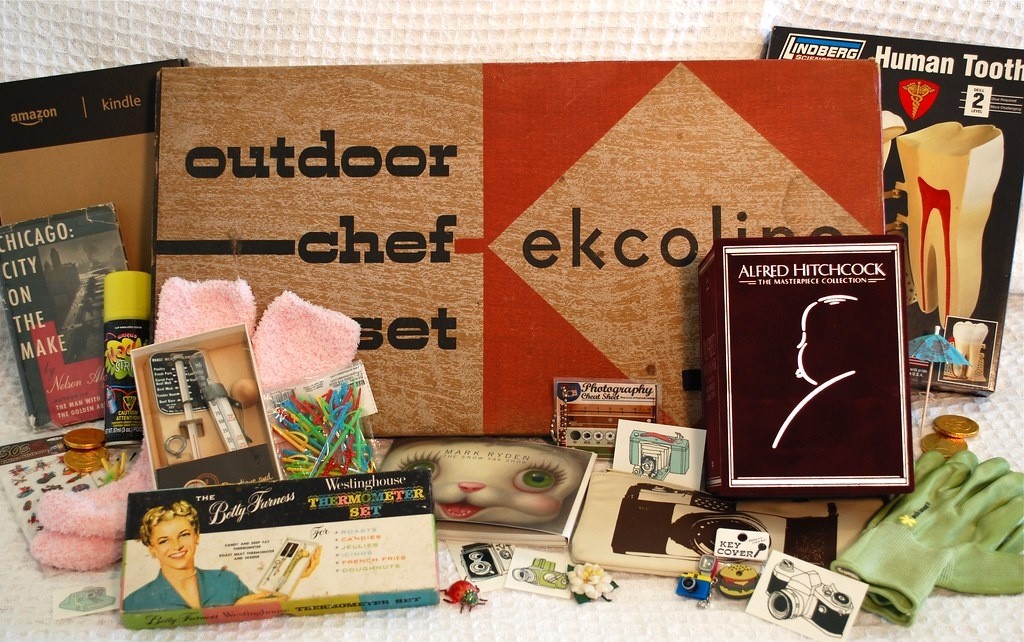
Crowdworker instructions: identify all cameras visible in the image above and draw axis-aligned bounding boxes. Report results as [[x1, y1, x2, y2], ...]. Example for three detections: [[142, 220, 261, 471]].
[[676, 576, 710, 599]]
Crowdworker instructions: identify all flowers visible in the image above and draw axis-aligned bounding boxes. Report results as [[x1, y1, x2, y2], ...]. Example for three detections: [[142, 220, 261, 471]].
[[564, 561, 614, 602]]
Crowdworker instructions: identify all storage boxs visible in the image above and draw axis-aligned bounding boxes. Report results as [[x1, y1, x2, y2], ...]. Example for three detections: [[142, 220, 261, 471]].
[[120, 468, 440, 630], [698, 234, 915, 497], [130, 321, 283, 490]]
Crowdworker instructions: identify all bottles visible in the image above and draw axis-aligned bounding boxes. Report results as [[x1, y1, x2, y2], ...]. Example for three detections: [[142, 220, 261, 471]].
[[103, 271, 155, 447]]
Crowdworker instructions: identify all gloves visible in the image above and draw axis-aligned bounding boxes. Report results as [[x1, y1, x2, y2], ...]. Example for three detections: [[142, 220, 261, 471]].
[[828, 448, 1024, 627]]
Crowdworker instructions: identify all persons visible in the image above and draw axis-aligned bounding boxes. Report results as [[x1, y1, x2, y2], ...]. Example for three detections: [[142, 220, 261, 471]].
[[121, 502, 320, 614]]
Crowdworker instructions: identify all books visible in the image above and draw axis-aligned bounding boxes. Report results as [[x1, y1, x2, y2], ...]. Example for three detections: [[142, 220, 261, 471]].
[[0, 203, 134, 432], [376, 435, 596, 549], [0, 59, 884, 456], [763, 25, 1024, 398]]
